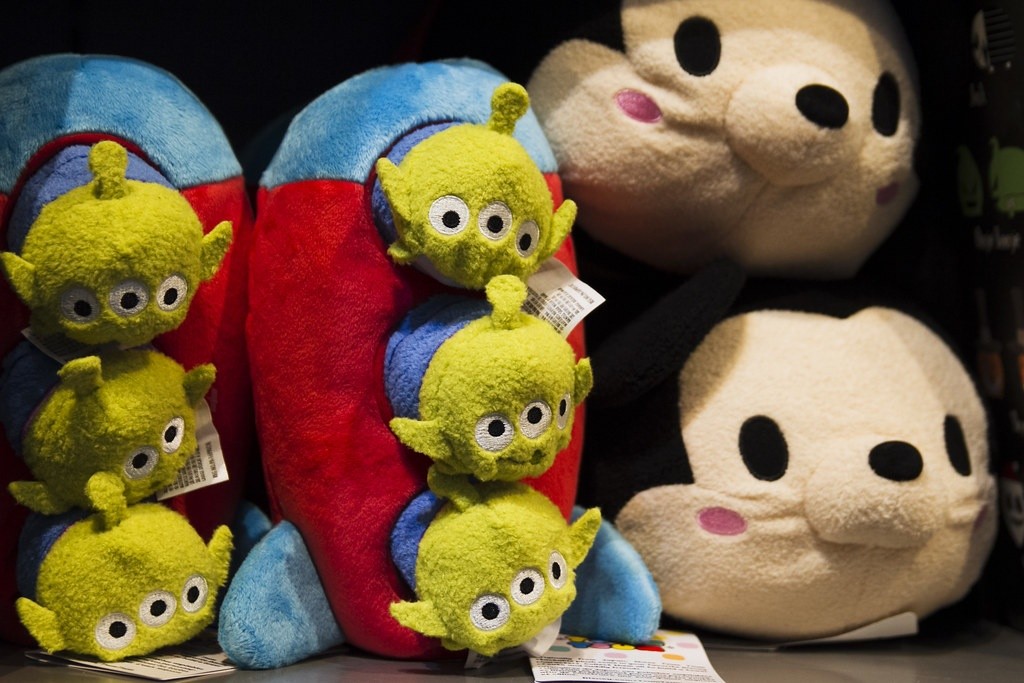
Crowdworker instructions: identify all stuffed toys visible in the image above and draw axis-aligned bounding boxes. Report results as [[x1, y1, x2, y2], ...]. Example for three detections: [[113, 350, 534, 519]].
[[574, 281, 1000, 639], [218, 57, 663, 667], [1, 50, 248, 658], [517, 0, 931, 280]]
